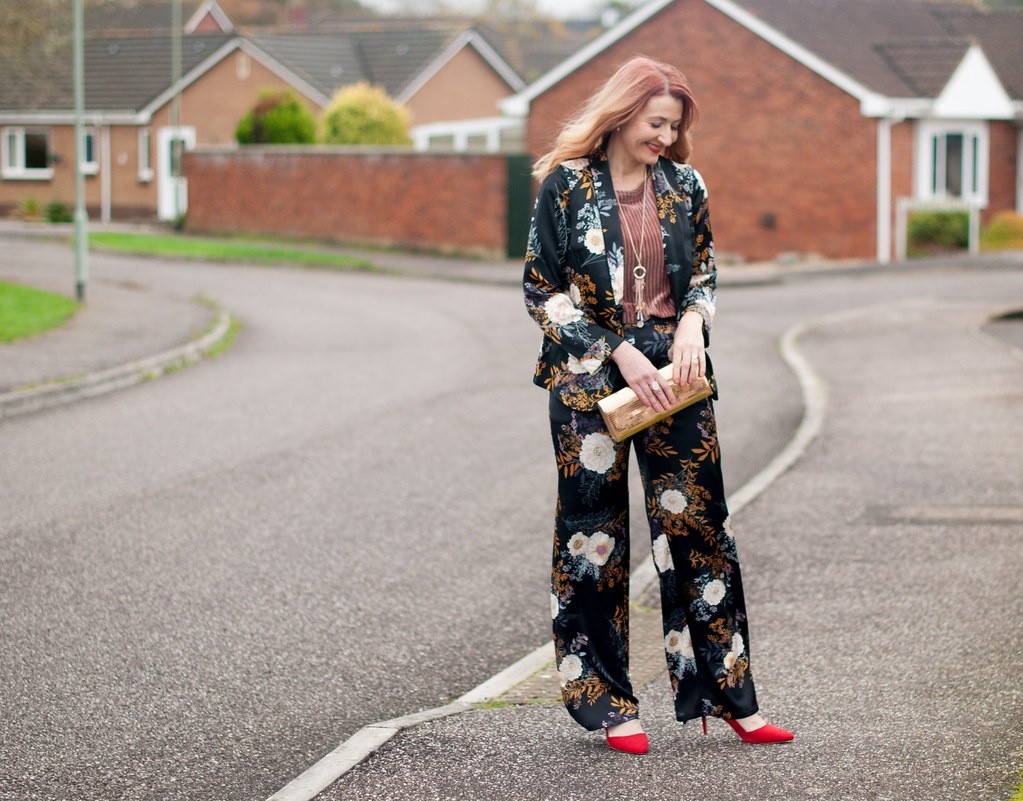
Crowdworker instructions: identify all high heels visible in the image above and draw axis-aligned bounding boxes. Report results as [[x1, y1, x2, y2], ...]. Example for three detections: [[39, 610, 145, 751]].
[[605, 723, 649, 755], [702, 715, 794, 743]]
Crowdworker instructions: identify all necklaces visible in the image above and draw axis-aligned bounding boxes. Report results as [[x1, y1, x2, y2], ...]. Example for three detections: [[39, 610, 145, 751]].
[[613, 166, 648, 322]]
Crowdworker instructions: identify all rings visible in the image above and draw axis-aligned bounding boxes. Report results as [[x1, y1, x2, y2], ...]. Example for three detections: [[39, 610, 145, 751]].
[[691, 355, 699, 360], [649, 381, 661, 391]]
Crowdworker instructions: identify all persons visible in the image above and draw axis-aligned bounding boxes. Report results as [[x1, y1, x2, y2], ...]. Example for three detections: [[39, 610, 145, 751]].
[[523, 56, 794, 754]]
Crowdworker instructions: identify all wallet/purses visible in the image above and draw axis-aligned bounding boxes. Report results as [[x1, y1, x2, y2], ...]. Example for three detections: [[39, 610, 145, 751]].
[[597, 361, 713, 443]]
